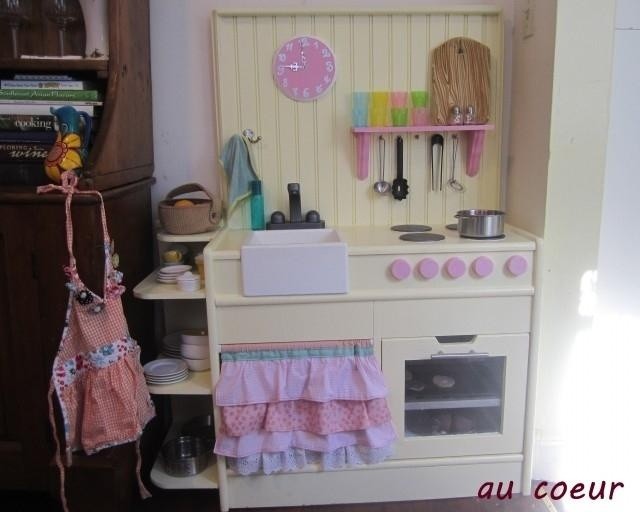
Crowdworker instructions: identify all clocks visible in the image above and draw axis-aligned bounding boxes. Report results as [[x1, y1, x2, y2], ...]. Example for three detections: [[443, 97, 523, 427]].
[[271, 35, 337, 102]]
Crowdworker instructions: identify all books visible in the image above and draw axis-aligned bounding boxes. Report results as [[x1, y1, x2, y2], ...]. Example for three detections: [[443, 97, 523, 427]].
[[0, 69, 106, 188]]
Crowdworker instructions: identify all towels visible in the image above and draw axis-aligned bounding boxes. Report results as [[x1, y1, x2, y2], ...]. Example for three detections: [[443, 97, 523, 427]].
[[215, 133, 264, 224]]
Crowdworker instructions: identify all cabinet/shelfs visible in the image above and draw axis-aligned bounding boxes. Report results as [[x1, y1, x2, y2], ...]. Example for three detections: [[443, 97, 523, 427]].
[[0, 179, 175, 509], [134, 219, 216, 493], [0, 0, 152, 188]]
